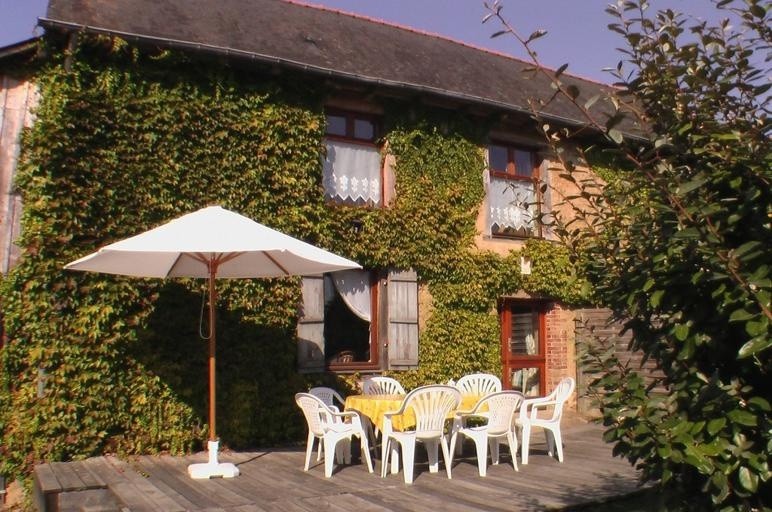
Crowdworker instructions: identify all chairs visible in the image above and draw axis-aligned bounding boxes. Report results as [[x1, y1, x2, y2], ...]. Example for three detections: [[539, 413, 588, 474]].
[[294, 392, 374, 479], [381, 384, 462, 484], [450, 391, 525, 476], [310, 387, 345, 462], [363, 378, 406, 394], [455, 375, 502, 394], [514, 377, 576, 466]]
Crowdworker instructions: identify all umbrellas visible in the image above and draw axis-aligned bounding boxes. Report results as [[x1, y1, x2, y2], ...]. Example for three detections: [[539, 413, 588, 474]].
[[60, 206, 363, 482]]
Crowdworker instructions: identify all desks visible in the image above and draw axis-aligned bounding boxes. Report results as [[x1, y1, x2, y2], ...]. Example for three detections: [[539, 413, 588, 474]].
[[342, 395, 503, 475]]
